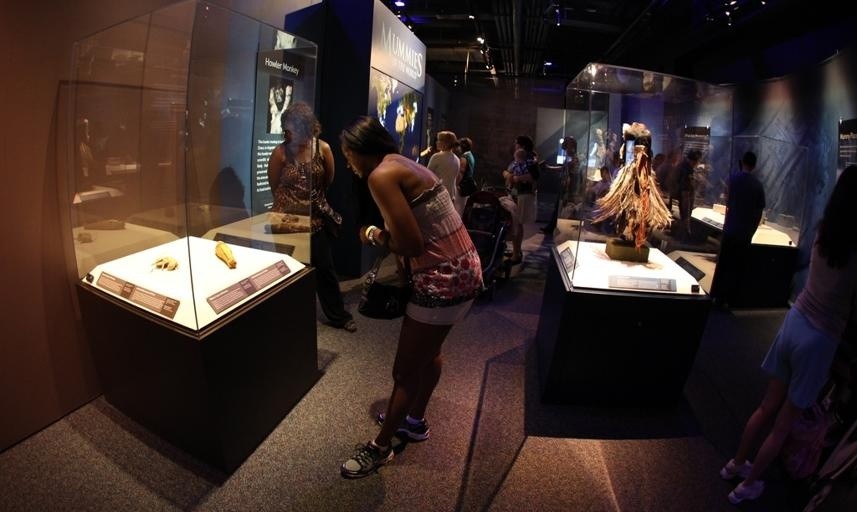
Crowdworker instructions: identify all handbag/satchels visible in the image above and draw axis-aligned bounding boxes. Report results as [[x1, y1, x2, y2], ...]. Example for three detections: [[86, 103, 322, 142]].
[[351, 243, 420, 318]]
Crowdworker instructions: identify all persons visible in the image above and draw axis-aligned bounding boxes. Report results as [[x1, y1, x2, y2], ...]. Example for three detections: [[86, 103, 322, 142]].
[[267, 101, 357, 333], [415, 131, 702, 266], [718, 152, 765, 300], [339, 115, 485, 479], [720, 164, 857, 503]]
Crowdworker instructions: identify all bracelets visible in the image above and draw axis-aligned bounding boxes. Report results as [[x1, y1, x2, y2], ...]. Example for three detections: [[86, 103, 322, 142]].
[[365, 225, 377, 246]]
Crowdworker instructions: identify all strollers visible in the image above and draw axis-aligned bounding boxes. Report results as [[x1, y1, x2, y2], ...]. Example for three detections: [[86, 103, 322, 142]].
[[462, 190, 514, 301]]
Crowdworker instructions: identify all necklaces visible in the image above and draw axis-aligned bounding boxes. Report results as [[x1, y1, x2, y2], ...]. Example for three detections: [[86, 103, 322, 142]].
[[294, 143, 307, 176]]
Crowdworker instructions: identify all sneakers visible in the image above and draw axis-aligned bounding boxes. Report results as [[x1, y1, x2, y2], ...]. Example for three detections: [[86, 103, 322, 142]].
[[721, 459, 771, 506], [378, 410, 432, 440], [341, 440, 396, 480], [335, 313, 360, 334], [510, 249, 526, 267]]
